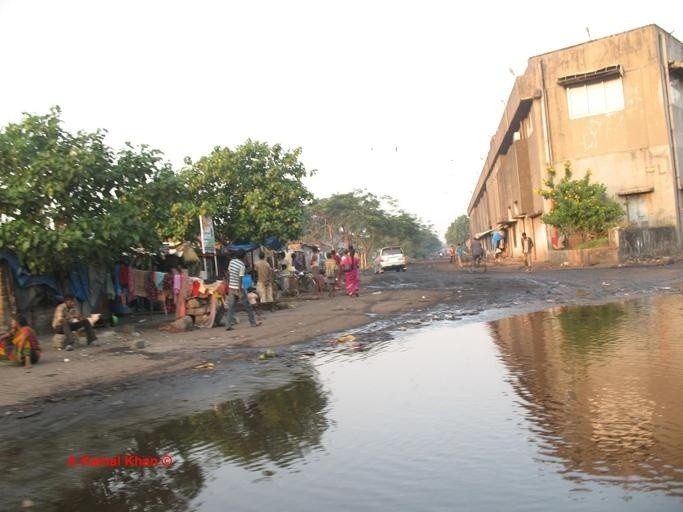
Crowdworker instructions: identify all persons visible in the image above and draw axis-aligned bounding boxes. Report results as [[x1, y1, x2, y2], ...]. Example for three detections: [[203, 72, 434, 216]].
[[457, 244, 462, 265], [521, 233, 533, 271], [472, 238, 483, 268], [225, 246, 360, 330], [52, 293, 100, 351], [450, 245, 455, 262], [495, 247, 502, 260], [0, 312, 41, 369]]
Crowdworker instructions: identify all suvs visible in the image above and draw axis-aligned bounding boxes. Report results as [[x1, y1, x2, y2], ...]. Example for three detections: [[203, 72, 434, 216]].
[[372, 245, 407, 274]]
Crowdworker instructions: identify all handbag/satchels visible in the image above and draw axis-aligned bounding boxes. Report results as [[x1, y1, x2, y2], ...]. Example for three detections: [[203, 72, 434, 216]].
[[343, 264, 352, 272]]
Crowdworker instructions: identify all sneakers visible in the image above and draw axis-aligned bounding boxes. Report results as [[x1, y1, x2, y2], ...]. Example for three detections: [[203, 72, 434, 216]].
[[92, 339, 100, 346], [66, 344, 74, 351]]
